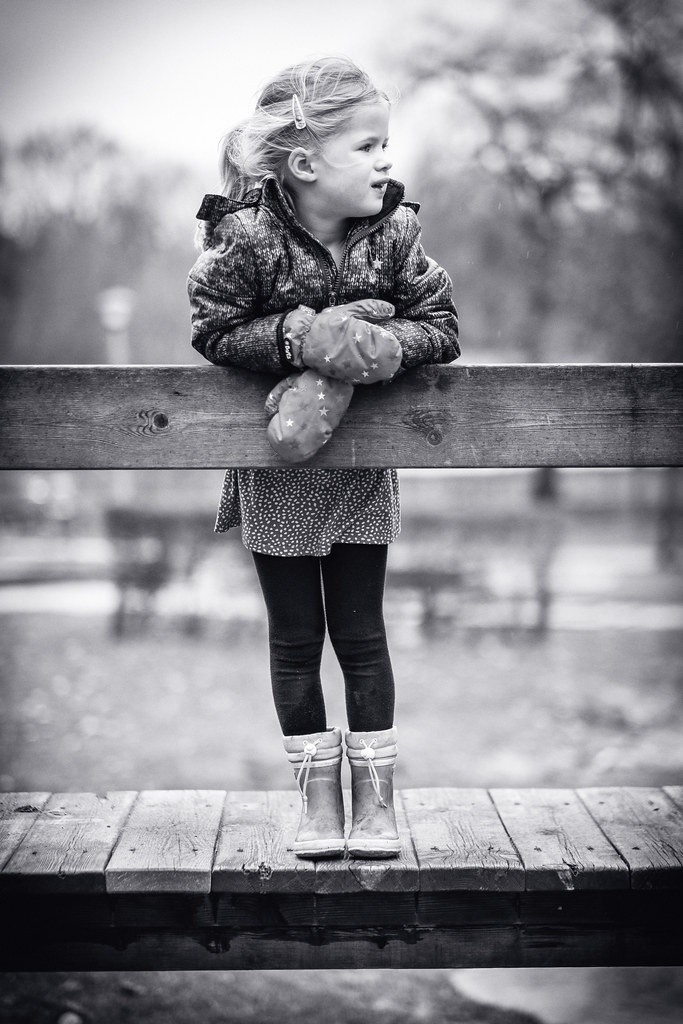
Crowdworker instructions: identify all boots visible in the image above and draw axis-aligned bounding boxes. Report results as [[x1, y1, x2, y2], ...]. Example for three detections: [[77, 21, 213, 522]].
[[344, 726, 402, 857], [282, 727, 347, 857]]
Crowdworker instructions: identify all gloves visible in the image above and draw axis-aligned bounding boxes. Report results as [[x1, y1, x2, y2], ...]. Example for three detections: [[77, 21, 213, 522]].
[[282, 298, 403, 385], [263, 369, 354, 464]]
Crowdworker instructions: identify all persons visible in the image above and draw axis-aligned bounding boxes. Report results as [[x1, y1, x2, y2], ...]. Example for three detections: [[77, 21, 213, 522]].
[[186, 57, 463, 860]]
